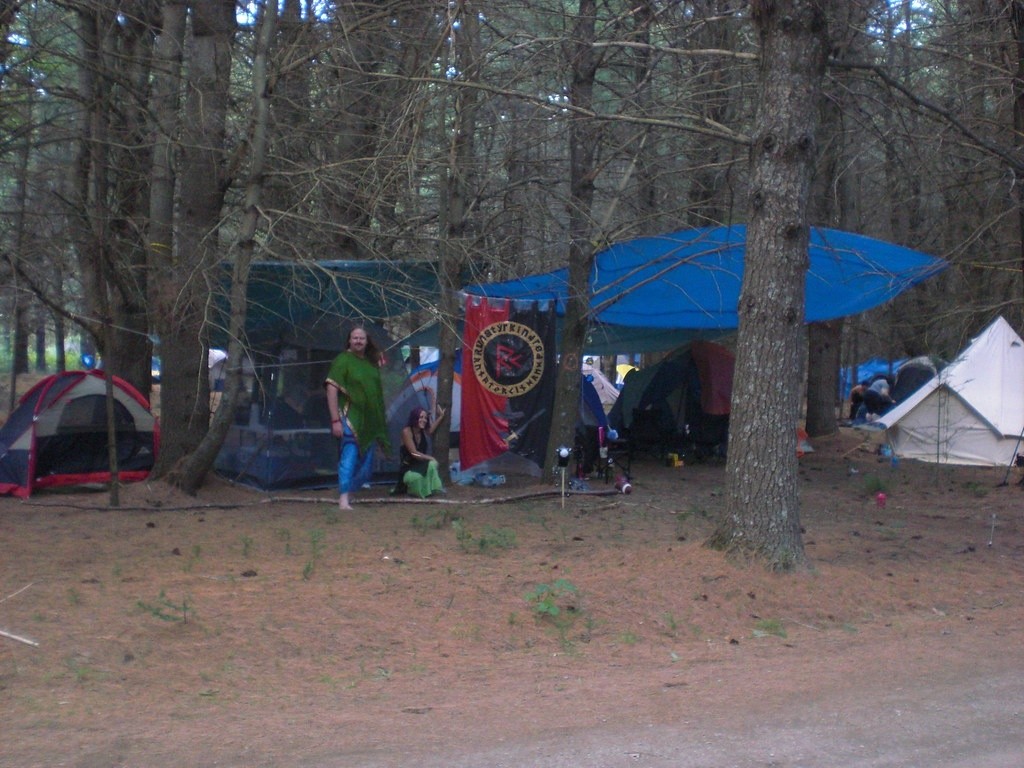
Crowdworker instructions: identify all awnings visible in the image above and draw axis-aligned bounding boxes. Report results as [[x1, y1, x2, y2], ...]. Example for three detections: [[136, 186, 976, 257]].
[[460, 221, 953, 330]]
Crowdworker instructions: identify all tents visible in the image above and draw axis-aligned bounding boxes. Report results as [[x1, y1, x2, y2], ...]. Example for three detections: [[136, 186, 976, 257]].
[[897, 355, 952, 390], [150, 334, 814, 491], [0, 366, 167, 500], [860, 316, 1024, 466]]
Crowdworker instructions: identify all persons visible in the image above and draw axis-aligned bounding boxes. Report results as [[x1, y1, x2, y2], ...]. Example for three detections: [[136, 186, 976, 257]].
[[848, 376, 897, 422], [325, 324, 389, 510], [390, 405, 446, 497]]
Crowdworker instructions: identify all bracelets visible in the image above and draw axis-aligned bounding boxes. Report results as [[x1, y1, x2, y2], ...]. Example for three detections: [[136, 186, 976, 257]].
[[332, 418, 341, 423]]
